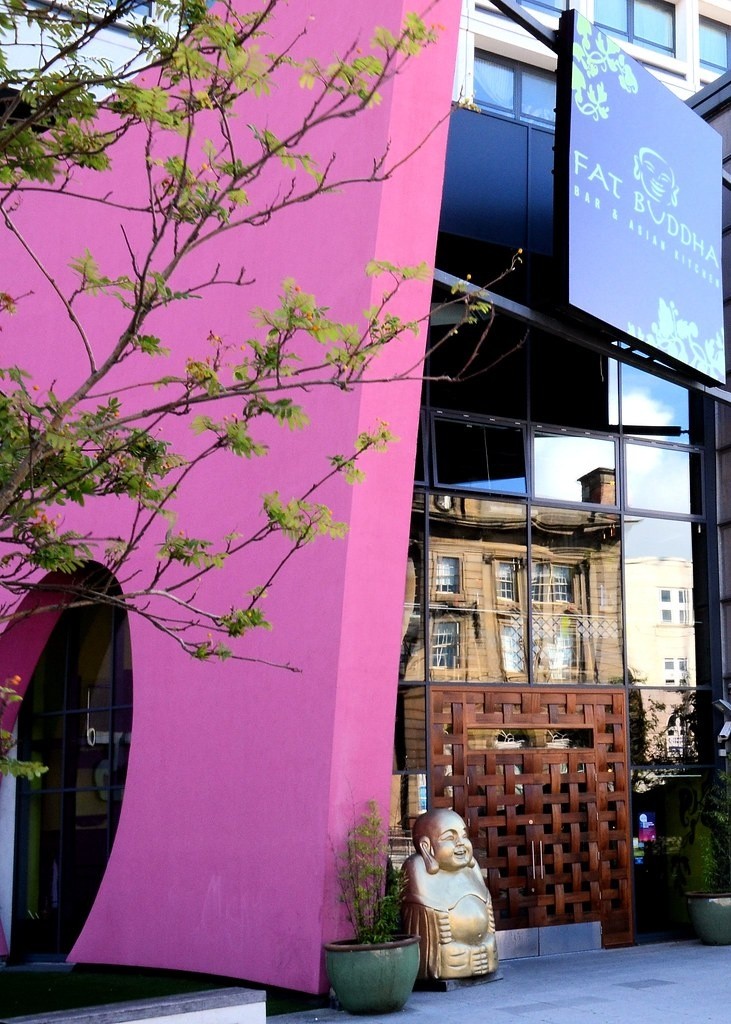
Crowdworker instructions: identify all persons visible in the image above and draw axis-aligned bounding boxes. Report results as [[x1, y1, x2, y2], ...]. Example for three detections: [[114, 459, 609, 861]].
[[398, 808, 500, 979]]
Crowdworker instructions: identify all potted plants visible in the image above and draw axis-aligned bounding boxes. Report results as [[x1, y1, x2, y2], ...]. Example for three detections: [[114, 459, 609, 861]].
[[319, 796, 422, 1017], [685, 768, 731, 946]]
